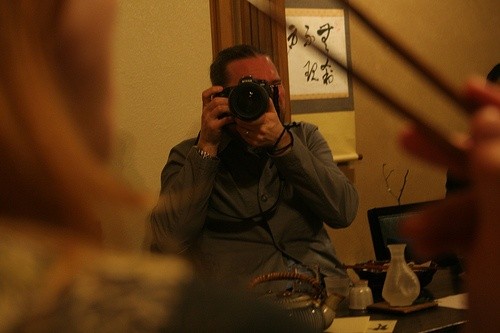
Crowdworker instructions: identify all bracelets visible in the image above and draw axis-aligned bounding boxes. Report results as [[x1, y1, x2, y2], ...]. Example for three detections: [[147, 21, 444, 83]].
[[268, 131, 294, 155], [191, 145, 217, 161]]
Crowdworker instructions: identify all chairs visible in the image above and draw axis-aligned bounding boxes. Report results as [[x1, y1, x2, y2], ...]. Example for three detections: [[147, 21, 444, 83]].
[[367, 199, 464, 295]]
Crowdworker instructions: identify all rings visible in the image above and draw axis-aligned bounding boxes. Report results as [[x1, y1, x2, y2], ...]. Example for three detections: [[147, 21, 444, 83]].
[[242, 129, 248, 136]]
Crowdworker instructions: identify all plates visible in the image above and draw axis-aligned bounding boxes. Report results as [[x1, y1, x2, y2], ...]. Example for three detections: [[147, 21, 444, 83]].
[[366, 300, 438, 314]]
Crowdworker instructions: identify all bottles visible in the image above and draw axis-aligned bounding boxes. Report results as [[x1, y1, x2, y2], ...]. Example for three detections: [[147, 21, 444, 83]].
[[382, 243, 420, 307], [348, 280, 374, 312]]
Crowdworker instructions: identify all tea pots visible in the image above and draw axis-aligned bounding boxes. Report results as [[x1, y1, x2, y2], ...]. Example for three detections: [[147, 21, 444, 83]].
[[244, 272, 347, 333]]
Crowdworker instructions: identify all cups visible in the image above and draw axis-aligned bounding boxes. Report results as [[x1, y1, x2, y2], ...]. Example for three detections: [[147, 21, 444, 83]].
[[322, 275, 347, 298]]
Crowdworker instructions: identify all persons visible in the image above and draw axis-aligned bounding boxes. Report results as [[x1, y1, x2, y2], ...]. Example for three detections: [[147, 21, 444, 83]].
[[400, 75, 500, 333], [150, 44, 359, 298]]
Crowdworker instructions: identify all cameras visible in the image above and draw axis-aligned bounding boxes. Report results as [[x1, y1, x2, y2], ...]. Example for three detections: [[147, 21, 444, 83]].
[[213, 75, 276, 128]]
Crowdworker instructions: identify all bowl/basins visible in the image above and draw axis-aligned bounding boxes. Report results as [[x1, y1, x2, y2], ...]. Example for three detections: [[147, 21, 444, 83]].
[[353, 260, 438, 291]]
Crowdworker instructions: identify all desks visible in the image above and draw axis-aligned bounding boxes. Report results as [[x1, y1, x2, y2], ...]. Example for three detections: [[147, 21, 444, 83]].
[[338, 263, 470, 333]]
